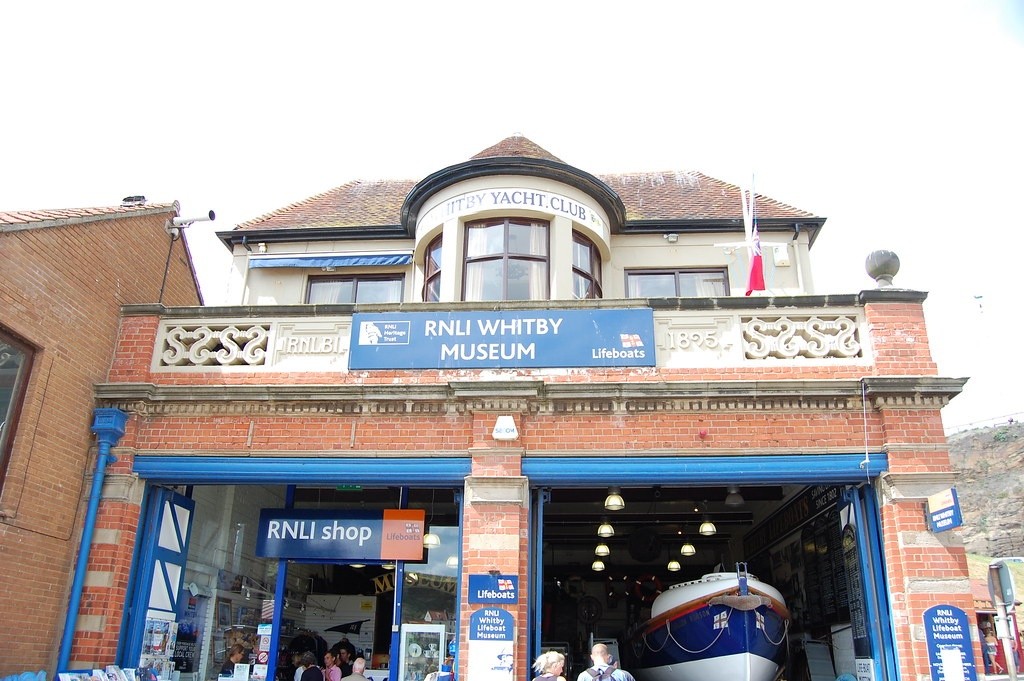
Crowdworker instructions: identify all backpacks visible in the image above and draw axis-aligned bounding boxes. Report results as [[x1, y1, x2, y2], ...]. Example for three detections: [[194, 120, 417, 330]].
[[585, 667, 617, 681]]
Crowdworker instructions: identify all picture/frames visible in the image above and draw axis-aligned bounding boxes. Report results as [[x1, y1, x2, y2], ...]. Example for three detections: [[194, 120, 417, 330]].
[[215, 596, 234, 634], [211, 635, 227, 668]]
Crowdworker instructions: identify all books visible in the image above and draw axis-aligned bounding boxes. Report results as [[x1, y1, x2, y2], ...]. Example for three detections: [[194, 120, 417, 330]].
[[58, 617, 178, 681]]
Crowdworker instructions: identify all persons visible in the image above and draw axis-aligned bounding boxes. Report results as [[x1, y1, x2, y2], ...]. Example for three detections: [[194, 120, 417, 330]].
[[529, 650, 567, 681], [979, 628, 1024, 675], [217, 638, 371, 681], [576, 643, 635, 681]]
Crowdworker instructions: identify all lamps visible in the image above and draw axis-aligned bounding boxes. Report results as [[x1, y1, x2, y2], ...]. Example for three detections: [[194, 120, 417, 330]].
[[592, 556, 605, 571], [667, 556, 681, 571], [604, 487, 625, 511], [597, 518, 616, 538], [680, 538, 696, 556], [594, 540, 610, 557], [698, 516, 717, 536]]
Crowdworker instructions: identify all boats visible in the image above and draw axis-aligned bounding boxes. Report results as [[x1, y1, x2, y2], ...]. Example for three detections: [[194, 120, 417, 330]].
[[629, 560, 794, 681]]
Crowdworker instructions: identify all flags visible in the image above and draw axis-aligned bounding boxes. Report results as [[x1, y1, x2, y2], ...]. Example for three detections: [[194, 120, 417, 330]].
[[745, 174, 765, 296]]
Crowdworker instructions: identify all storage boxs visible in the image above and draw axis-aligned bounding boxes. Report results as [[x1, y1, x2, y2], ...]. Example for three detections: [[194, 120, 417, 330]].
[[397, 623, 448, 681]]
